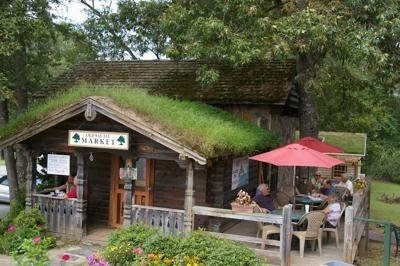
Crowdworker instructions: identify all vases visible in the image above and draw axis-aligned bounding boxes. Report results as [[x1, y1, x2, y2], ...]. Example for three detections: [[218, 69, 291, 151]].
[[231, 202, 254, 215]]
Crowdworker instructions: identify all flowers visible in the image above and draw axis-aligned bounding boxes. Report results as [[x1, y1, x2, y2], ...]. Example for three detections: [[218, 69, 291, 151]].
[[353, 179, 367, 187], [235, 188, 252, 205]]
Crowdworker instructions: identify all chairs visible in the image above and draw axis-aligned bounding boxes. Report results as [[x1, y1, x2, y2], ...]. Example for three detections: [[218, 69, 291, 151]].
[[254, 175, 356, 259]]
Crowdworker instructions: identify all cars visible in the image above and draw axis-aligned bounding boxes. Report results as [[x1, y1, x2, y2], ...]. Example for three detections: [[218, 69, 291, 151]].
[[0, 169, 47, 204]]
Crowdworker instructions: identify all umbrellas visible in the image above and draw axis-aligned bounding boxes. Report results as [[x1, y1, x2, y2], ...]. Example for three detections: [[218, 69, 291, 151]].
[[293, 136, 344, 181], [245, 143, 344, 214]]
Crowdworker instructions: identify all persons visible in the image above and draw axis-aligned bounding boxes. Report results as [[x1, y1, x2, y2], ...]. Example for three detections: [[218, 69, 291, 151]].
[[294, 171, 354, 231], [252, 183, 282, 240], [54, 175, 73, 194], [64, 176, 77, 198]]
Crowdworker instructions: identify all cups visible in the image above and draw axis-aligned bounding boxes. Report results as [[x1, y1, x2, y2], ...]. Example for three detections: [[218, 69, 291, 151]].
[[304, 204, 310, 213]]
